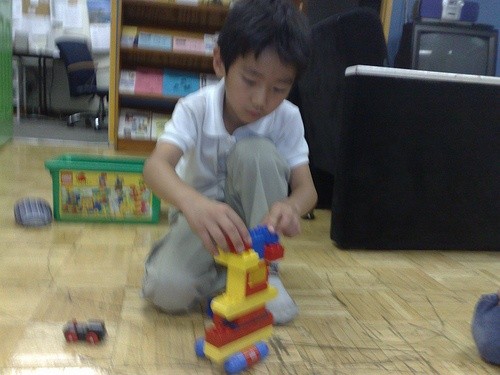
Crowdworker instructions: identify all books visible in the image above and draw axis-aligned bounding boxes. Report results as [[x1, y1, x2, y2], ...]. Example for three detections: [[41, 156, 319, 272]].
[[117, 24, 220, 143]]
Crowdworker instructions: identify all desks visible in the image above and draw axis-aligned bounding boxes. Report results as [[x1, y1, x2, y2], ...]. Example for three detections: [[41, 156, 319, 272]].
[[13, 49, 61, 119], [329, 64, 500, 251]]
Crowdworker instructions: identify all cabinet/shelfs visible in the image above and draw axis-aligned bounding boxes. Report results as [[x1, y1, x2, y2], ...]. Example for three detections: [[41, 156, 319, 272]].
[[108, 0, 229, 150]]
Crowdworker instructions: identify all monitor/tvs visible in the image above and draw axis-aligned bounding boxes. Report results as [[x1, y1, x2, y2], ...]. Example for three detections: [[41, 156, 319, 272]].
[[394, 22, 498, 76]]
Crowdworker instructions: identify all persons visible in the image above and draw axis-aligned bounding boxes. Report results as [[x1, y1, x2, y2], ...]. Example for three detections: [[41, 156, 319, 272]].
[[139, 0, 320, 325], [471, 289, 500, 367]]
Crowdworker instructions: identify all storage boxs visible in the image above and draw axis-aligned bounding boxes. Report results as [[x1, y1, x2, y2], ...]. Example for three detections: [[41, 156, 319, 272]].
[[14, 29, 48, 54], [44, 153, 160, 224]]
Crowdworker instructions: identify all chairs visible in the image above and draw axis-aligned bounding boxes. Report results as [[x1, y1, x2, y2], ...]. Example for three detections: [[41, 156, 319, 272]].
[[55, 36, 109, 132]]
